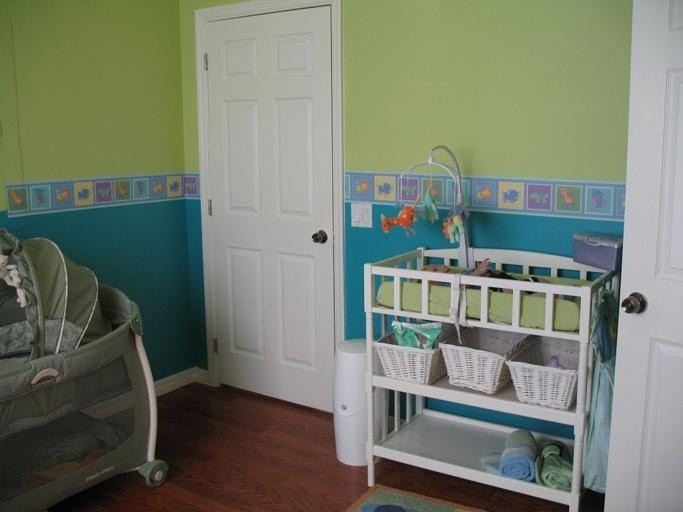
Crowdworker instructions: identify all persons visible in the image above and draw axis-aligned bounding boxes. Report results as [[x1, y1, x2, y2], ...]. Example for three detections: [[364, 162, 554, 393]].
[[417, 256, 550, 299]]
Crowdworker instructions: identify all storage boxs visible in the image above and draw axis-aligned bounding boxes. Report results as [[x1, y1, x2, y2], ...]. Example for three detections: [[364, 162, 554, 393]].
[[571, 233, 623, 272]]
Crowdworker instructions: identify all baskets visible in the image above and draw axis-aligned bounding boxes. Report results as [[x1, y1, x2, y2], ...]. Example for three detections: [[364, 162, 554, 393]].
[[438, 328, 530, 395], [505, 335, 578, 410], [373, 333, 444, 385]]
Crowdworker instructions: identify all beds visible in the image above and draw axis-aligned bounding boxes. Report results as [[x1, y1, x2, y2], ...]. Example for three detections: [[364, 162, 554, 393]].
[[363, 245, 622, 512]]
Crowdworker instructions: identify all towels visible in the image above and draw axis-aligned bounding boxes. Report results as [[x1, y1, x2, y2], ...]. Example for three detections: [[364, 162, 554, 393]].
[[498, 430, 540, 483], [534, 436, 573, 492]]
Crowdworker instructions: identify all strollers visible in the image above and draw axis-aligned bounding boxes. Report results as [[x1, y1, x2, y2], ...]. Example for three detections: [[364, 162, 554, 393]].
[[0, 227, 168, 511]]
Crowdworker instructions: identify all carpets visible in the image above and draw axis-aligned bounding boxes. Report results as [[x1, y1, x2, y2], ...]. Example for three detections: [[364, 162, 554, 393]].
[[345, 483, 487, 512]]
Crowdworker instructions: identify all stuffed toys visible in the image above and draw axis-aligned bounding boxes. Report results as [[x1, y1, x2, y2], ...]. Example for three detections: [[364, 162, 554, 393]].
[[378, 203, 421, 238], [420, 188, 440, 227], [440, 206, 470, 246]]
